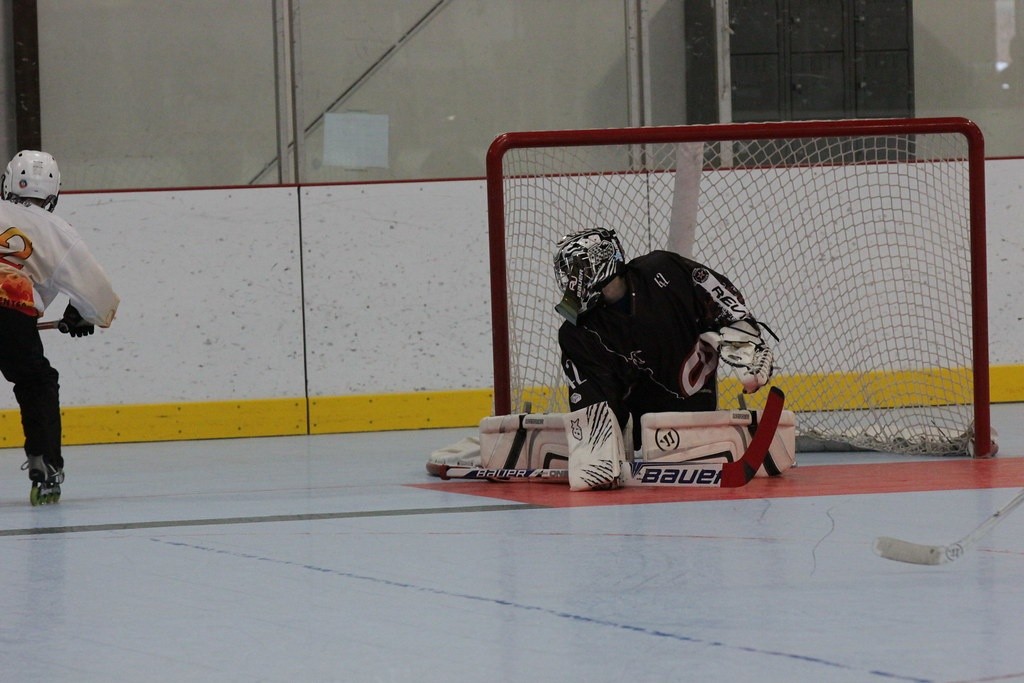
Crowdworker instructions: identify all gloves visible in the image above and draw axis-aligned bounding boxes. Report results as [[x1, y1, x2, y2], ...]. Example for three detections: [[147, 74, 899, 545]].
[[58, 300, 95, 338]]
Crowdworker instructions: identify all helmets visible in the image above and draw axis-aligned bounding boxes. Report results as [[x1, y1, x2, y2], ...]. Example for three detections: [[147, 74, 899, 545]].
[[0, 150, 61, 213], [554, 228, 625, 314]]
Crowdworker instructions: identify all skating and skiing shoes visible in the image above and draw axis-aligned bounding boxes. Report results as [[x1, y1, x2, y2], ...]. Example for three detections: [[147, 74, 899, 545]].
[[20, 456, 65, 505]]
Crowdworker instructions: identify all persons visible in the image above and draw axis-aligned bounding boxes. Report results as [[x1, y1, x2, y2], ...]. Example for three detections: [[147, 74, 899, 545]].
[[0, 150, 121, 505], [426, 227, 796, 492]]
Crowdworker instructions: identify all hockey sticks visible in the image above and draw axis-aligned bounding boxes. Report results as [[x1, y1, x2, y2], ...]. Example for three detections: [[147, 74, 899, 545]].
[[439, 385, 786, 490], [36, 320, 60, 330]]
